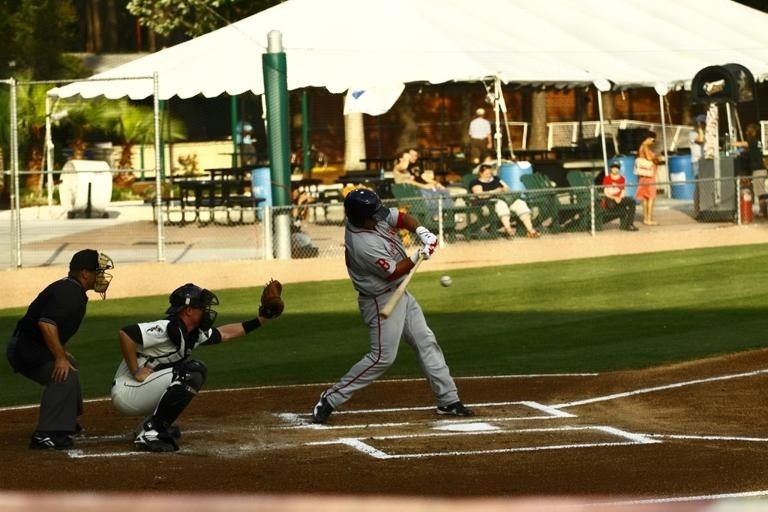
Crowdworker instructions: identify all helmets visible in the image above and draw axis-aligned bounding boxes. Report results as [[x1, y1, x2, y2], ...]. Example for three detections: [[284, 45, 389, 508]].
[[343, 189, 391, 229]]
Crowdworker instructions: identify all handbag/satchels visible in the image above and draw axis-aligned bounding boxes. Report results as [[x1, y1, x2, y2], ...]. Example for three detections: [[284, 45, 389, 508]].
[[633, 157, 661, 179]]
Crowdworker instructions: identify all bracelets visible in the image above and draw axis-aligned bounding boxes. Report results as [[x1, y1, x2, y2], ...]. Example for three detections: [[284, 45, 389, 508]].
[[132, 367, 140, 377], [410, 252, 419, 265], [242, 317, 262, 334]]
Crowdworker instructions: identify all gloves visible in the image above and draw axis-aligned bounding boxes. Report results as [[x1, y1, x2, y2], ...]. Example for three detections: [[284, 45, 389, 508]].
[[410, 227, 438, 266]]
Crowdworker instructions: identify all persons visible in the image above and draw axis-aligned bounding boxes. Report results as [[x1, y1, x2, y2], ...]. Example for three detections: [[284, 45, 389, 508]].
[[110, 277, 285, 452], [602, 162, 638, 231], [235, 107, 259, 167], [7, 249, 114, 451], [635, 134, 659, 225], [689, 114, 706, 179], [290, 111, 316, 173], [312, 188, 474, 424], [392, 108, 540, 239], [729, 123, 764, 176]]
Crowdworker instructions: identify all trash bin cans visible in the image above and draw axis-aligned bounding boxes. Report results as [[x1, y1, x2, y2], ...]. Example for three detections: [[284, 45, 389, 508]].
[[499, 161, 533, 200]]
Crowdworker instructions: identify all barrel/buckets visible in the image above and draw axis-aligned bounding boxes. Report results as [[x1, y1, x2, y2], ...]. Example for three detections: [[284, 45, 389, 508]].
[[499, 161, 533, 195]]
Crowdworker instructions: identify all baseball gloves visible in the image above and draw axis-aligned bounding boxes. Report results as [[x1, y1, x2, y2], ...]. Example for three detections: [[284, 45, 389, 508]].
[[261, 280, 284, 317]]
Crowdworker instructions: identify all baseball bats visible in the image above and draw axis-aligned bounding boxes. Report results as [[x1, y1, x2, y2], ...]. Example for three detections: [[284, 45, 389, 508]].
[[380, 254, 423, 319]]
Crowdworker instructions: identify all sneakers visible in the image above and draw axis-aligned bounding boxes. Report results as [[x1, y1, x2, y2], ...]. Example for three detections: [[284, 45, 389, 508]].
[[28, 432, 73, 450], [620, 225, 638, 232], [524, 230, 539, 238], [435, 400, 476, 421], [504, 231, 514, 239], [642, 219, 659, 226], [130, 421, 182, 454], [311, 388, 331, 423]]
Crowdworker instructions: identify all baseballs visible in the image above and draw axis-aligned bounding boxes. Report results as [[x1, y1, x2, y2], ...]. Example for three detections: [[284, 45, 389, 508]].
[[441, 276, 451, 286]]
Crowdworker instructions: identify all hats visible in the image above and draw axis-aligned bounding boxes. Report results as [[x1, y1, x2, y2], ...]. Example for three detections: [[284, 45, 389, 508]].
[[608, 161, 621, 168], [643, 131, 657, 139], [163, 282, 214, 314], [69, 248, 111, 273]]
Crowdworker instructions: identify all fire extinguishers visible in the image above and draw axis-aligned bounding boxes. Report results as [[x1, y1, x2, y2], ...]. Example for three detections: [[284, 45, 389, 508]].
[[740, 178, 752, 224]]
